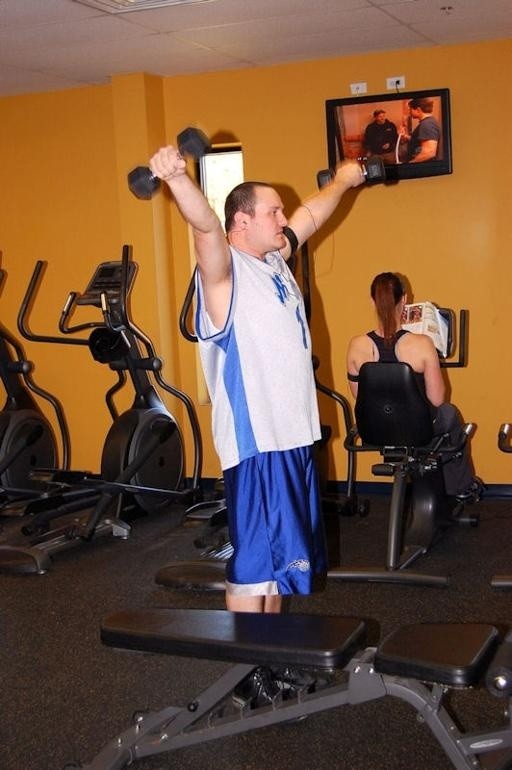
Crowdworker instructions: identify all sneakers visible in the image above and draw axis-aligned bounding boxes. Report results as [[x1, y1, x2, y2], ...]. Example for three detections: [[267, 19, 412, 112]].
[[232, 666, 309, 725], [455, 478, 482, 504], [273, 664, 336, 693]]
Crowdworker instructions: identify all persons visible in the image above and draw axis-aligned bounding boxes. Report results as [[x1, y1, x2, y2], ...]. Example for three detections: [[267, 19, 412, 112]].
[[149, 145, 366, 728], [361, 109, 398, 165], [346, 272, 483, 504], [400, 98, 440, 165]]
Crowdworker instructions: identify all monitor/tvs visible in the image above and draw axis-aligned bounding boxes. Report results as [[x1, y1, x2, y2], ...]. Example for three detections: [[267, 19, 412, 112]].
[[325, 87, 453, 182], [76, 260, 139, 306]]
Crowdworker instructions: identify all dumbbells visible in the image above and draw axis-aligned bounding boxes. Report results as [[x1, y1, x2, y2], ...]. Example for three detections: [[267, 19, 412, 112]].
[[128, 127, 212, 200], [317, 157, 386, 190]]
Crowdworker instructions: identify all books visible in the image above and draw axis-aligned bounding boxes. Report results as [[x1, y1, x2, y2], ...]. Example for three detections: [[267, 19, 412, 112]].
[[399, 301, 448, 358]]
